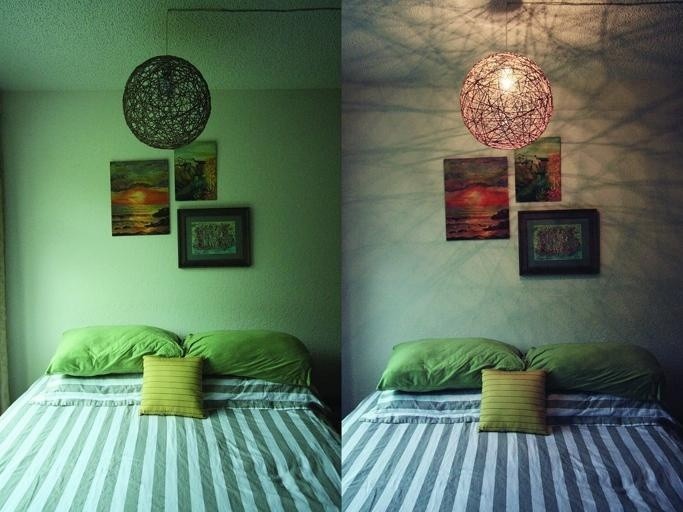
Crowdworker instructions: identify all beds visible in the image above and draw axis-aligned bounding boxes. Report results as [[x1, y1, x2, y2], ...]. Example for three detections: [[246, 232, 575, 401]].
[[0, 368, 341, 511], [342, 388, 683, 511]]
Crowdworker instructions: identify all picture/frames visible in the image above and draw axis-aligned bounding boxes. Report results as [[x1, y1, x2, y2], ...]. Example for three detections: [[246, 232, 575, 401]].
[[178, 208, 250, 267], [518, 209, 600, 276]]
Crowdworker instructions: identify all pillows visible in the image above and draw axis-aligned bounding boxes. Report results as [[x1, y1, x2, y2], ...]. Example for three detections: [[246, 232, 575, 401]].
[[47, 321, 315, 419], [377, 336, 667, 434]]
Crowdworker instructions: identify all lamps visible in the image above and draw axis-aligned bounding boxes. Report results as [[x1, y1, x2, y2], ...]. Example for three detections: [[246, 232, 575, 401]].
[[460, 0, 554, 152], [122, 0, 212, 151]]
